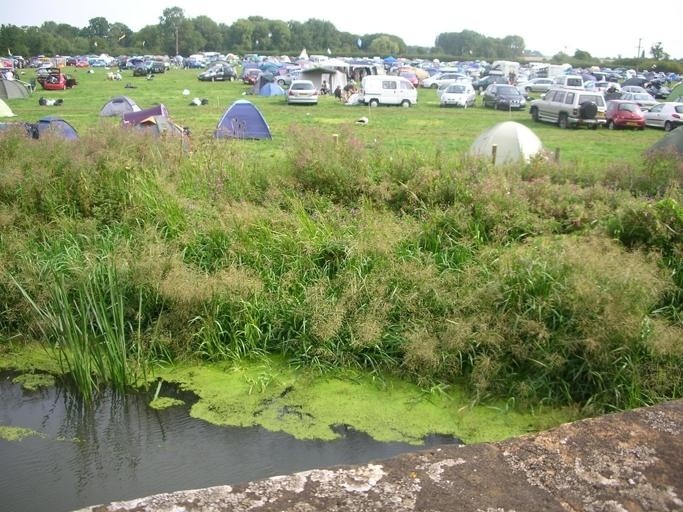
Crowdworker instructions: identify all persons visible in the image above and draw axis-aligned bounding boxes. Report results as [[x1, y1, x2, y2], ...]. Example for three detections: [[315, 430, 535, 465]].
[[107, 71, 122, 81], [320, 72, 358, 103]]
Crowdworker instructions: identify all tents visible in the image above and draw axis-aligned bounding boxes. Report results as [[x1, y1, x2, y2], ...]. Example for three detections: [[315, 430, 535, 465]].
[[214, 99, 271, 141], [467, 121, 548, 169], [259, 82, 284, 97], [0, 71, 79, 148], [641, 121, 683, 161], [99, 95, 188, 152]]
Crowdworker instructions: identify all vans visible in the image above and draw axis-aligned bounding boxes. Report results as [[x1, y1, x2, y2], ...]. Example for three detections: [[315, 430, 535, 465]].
[[143, 61, 164, 74], [360, 74, 417, 107]]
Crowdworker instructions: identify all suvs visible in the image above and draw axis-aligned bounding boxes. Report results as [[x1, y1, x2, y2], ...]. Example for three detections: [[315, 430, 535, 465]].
[[132, 64, 147, 76]]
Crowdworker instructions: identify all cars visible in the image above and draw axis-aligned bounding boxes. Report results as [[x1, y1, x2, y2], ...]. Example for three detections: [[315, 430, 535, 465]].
[[420, 61, 683, 131], [275, 69, 302, 86], [242, 69, 264, 84], [286, 79, 318, 104], [184, 57, 211, 68], [37, 67, 77, 90], [197, 66, 237, 82], [64, 55, 170, 70]]
